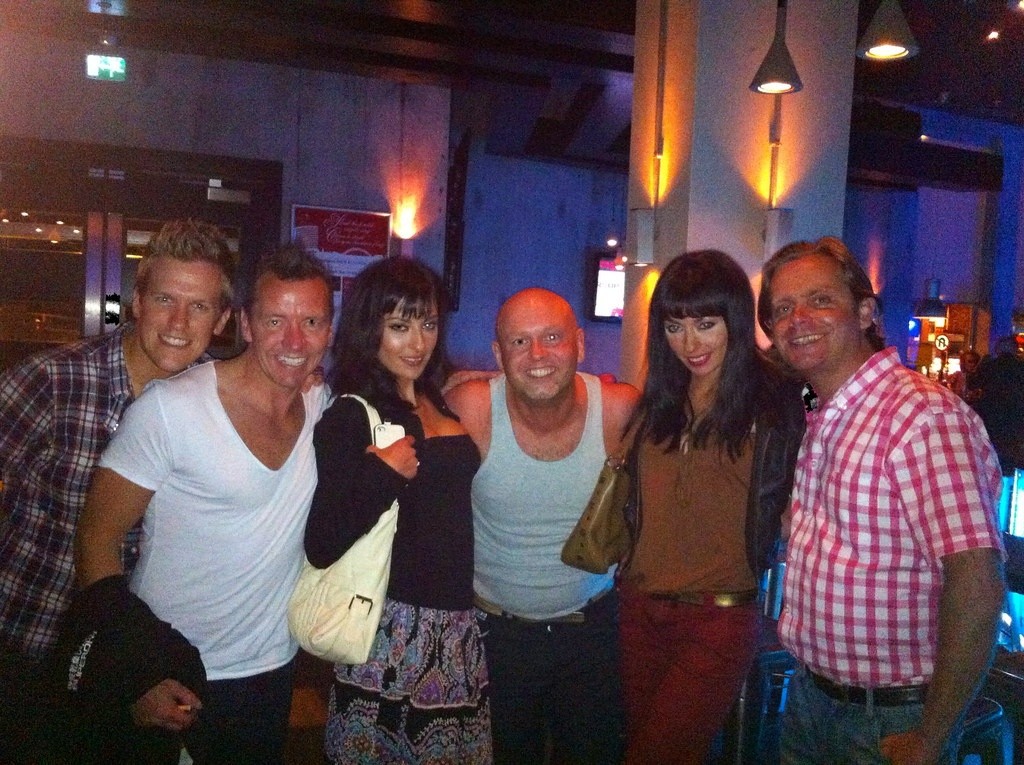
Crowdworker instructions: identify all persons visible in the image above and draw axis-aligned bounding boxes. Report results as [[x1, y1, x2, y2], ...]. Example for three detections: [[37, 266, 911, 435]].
[[755, 236, 1009, 765], [73, 246, 336, 765], [944, 337, 1024, 478], [301, 256, 496, 764], [601, 250, 807, 765], [439, 287, 644, 764], [0, 218, 239, 765]]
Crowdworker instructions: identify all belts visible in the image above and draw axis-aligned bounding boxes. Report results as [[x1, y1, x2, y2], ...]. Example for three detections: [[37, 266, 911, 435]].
[[802, 665, 929, 708], [645, 589, 763, 608], [471, 598, 612, 626]]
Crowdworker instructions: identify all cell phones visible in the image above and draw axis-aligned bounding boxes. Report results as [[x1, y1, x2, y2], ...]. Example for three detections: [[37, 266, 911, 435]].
[[375, 424, 406, 448]]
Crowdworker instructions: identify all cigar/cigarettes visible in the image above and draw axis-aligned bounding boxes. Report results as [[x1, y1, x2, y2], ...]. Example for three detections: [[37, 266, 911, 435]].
[[177, 704, 191, 712]]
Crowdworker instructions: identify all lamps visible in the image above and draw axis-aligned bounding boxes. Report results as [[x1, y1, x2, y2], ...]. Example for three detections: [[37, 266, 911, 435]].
[[854, 1, 921, 62], [748, 0, 804, 96]]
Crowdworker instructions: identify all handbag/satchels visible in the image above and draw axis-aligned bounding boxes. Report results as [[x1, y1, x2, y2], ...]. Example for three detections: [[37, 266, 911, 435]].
[[286, 393, 407, 665], [558, 407, 651, 577]]
